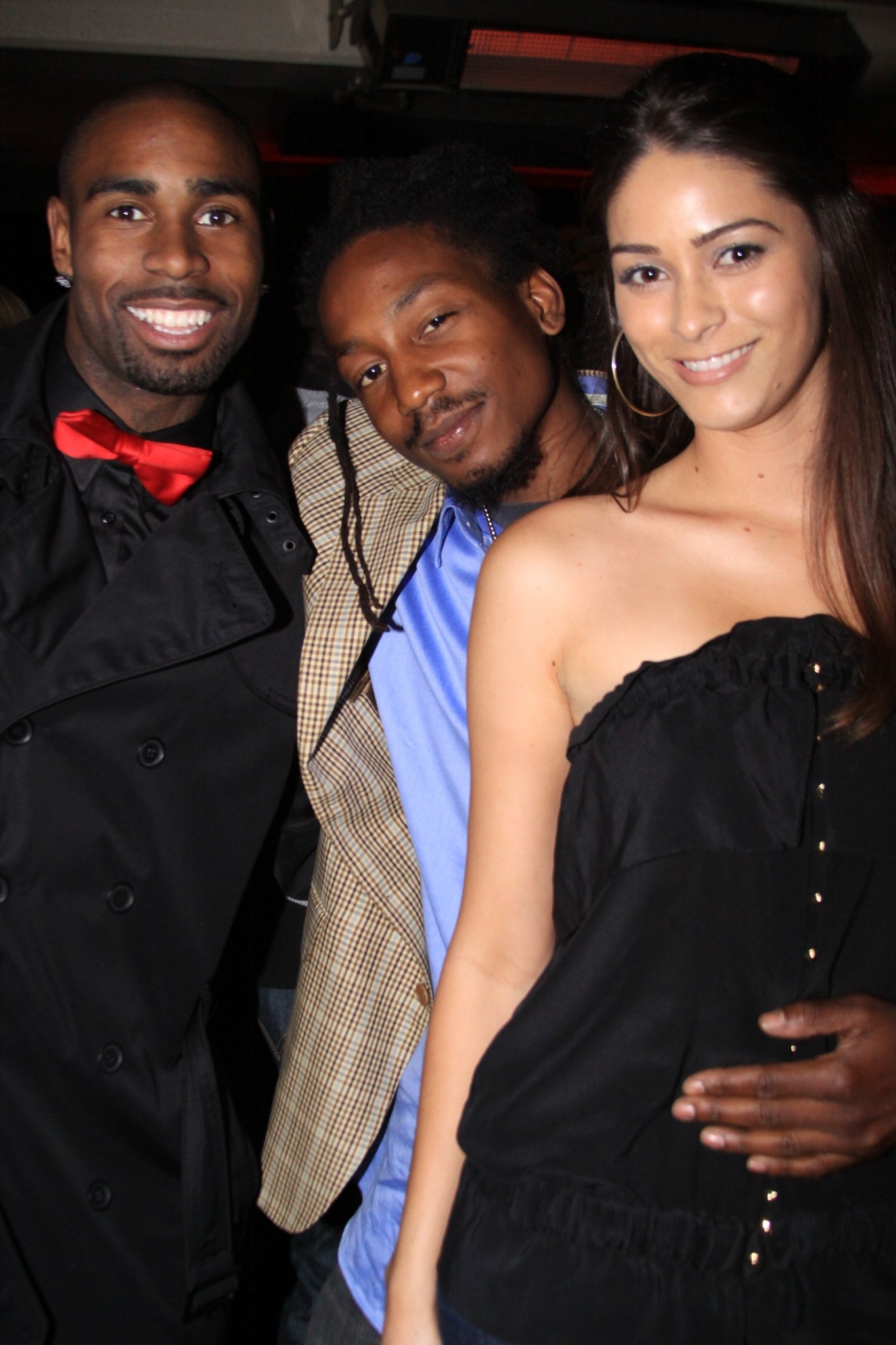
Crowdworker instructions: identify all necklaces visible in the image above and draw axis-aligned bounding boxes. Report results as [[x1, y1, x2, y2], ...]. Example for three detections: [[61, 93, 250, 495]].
[[475, 405, 612, 541]]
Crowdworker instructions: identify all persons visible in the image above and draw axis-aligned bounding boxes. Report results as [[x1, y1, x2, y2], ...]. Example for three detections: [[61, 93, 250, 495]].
[[255, 161, 896, 1345], [380, 49, 896, 1345], [0, 58, 315, 1345]]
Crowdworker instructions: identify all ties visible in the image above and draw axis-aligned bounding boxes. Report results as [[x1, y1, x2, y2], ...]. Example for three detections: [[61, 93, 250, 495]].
[[55, 410, 214, 507]]
